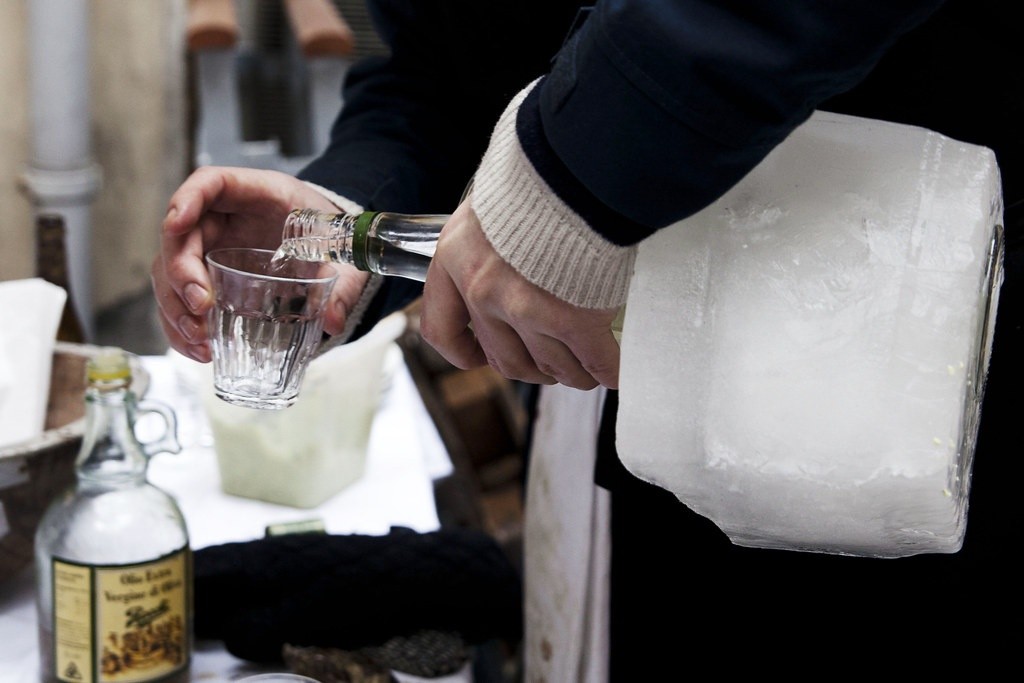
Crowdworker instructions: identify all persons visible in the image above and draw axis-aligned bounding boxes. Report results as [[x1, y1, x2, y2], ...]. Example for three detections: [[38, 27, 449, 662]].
[[145, 0, 1024, 683]]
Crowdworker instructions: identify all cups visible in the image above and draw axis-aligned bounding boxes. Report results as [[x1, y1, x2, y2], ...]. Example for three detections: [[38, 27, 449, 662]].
[[203, 248, 339, 410]]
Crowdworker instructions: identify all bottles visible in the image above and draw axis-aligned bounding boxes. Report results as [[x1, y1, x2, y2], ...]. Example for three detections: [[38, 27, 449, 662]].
[[29, 213, 88, 344], [35, 350, 194, 683], [280, 209, 624, 331]]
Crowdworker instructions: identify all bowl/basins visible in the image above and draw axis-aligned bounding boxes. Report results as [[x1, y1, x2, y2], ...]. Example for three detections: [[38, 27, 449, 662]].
[[1, 342, 154, 609]]
